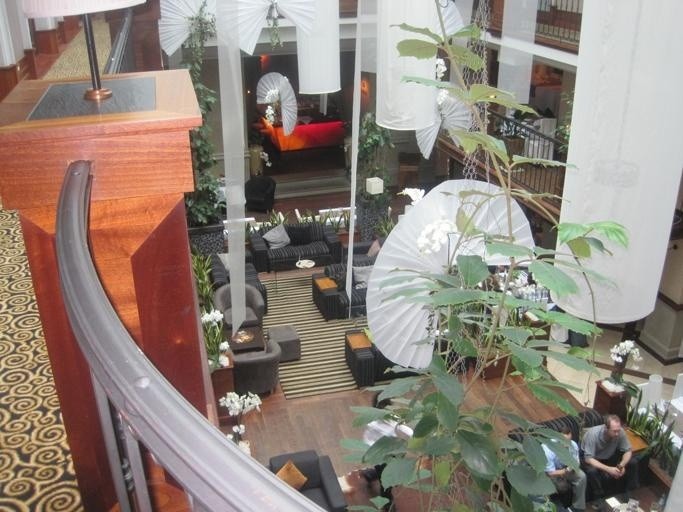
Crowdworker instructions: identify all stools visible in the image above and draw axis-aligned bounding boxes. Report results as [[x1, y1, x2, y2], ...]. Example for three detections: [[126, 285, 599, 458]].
[[268, 325, 300, 363], [399, 151, 421, 191]]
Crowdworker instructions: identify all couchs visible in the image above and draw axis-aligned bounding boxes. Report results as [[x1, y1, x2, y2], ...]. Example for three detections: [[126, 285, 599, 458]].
[[345, 202, 553, 390], [243, 99, 351, 220], [211, 255, 268, 314], [498, 410, 651, 508], [250, 222, 342, 272], [311, 260, 375, 321]]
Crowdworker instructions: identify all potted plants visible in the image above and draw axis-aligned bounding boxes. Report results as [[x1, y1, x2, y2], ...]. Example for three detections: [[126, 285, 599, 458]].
[[201, 311, 236, 394]]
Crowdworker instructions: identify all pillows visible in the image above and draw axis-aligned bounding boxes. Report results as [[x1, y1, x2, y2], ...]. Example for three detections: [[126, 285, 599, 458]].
[[261, 222, 290, 251], [287, 226, 312, 246], [191, 231, 223, 255], [276, 460, 308, 491], [218, 253, 230, 277]]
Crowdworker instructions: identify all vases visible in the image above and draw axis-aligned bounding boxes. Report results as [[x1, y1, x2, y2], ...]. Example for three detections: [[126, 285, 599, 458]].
[[594, 380, 634, 422]]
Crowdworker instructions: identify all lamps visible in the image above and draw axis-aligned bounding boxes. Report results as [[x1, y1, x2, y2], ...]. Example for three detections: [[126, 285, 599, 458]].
[[21, 0, 145, 101]]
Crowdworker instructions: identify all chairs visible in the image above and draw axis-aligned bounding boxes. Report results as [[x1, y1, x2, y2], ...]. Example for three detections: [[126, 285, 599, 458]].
[[233, 340, 281, 394], [484, 101, 555, 175], [264, 449, 347, 512], [214, 283, 264, 331]]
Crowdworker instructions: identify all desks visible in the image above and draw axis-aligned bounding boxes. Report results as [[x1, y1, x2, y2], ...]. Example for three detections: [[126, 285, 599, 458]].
[[223, 327, 264, 352]]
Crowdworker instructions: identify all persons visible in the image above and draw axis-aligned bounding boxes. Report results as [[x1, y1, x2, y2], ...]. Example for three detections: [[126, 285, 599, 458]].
[[361, 389, 413, 512], [578, 413, 642, 501], [536, 423, 589, 512]]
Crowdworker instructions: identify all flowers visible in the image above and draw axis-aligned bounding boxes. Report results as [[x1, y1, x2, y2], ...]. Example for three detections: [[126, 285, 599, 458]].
[[603, 338, 643, 398]]
